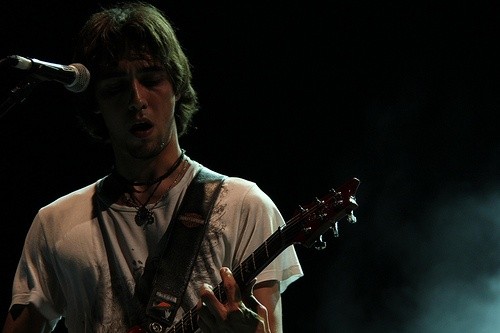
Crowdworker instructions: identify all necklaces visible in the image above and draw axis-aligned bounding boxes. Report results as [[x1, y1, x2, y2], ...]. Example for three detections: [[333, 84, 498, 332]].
[[112, 148, 189, 227]]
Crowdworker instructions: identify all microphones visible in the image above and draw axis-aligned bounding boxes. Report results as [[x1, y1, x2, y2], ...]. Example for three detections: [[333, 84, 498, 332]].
[[11, 54, 90, 93]]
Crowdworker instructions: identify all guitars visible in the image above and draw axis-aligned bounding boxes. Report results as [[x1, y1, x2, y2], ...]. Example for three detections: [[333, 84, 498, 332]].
[[164, 177, 360, 333]]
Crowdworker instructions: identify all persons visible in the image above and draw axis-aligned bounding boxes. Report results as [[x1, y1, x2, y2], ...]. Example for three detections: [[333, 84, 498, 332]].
[[0, 3, 304, 332]]
